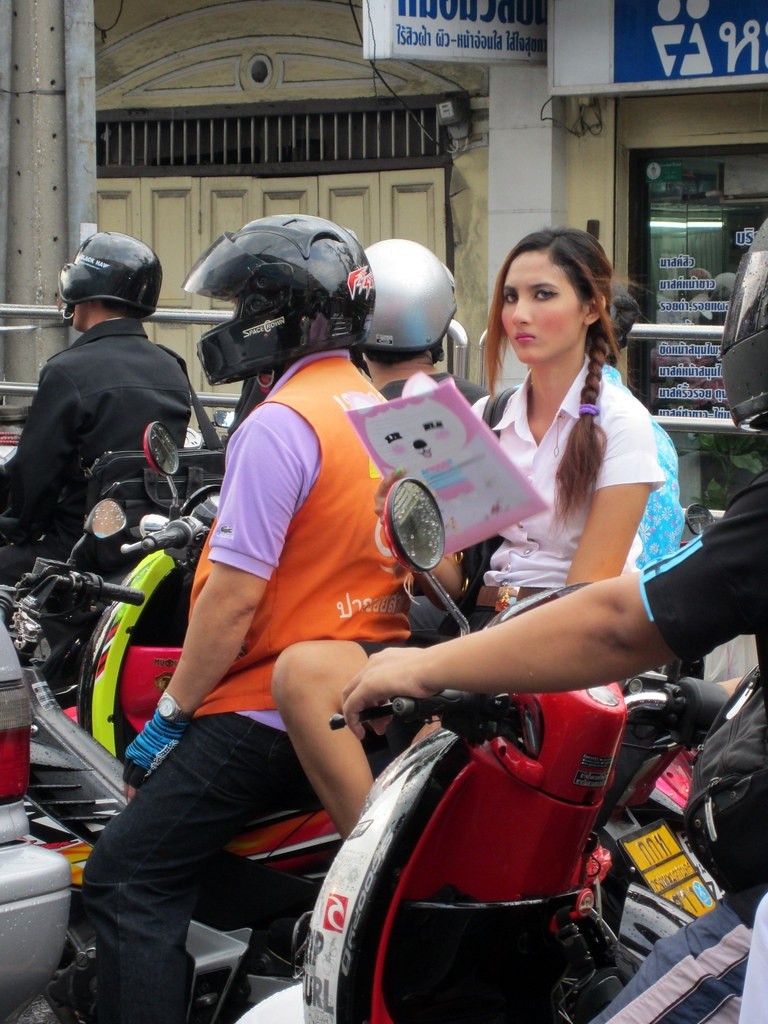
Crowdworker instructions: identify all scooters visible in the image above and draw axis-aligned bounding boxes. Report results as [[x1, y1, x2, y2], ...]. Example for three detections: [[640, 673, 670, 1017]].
[[302, 477, 729, 1024], [78, 420, 699, 980], [0, 497, 137, 901]]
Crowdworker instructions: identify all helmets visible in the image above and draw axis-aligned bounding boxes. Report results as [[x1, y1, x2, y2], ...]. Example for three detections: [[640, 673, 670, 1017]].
[[361, 239, 458, 353], [721, 217, 768, 435], [182, 214, 376, 386], [58, 232, 162, 313]]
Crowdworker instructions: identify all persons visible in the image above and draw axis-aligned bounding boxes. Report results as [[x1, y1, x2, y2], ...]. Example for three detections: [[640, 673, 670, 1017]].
[[343, 220, 768, 1024], [1, 229, 196, 582], [89, 214, 684, 1024]]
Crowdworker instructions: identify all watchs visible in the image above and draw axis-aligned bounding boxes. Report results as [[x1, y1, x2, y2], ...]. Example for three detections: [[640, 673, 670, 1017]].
[[157, 690, 194, 723]]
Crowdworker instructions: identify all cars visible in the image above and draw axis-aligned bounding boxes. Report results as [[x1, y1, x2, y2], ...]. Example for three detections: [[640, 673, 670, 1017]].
[[0, 611, 72, 1000]]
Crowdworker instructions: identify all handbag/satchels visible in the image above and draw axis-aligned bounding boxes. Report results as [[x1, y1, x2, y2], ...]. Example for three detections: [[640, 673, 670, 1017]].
[[682, 666, 768, 931]]
